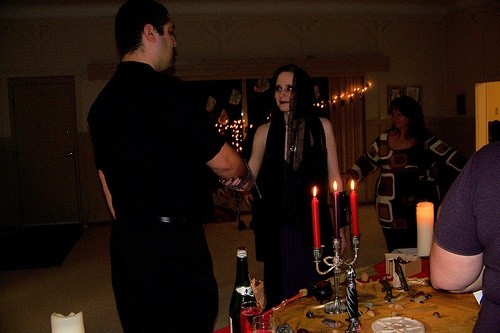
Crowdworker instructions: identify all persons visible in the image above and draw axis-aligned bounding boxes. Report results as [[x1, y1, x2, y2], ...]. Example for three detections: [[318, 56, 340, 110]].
[[217, 64, 343, 309], [430, 145, 500, 333], [88, 0, 248, 333], [344, 96, 464, 254]]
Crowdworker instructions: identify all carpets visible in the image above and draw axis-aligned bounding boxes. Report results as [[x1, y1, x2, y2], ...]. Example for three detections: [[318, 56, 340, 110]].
[[0, 224, 84, 270]]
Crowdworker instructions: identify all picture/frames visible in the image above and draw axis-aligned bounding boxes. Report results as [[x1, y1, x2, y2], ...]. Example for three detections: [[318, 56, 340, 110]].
[[387, 85, 424, 113]]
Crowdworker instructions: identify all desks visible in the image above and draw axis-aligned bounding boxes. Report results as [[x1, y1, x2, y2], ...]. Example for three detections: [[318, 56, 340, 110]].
[[218, 261, 481, 333]]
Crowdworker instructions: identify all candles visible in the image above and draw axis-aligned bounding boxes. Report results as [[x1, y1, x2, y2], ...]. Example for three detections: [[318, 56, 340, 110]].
[[415, 201, 434, 257], [313, 185, 321, 249], [333, 180, 341, 239], [349, 181, 358, 240]]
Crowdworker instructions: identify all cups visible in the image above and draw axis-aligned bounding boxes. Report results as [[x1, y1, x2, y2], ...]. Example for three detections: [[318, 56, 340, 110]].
[[239, 300, 262, 333]]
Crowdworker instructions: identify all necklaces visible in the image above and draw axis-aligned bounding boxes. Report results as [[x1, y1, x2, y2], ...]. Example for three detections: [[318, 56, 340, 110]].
[[290, 145, 297, 152]]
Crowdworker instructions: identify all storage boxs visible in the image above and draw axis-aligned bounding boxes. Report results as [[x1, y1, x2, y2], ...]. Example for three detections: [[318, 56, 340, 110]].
[[384, 247, 431, 279]]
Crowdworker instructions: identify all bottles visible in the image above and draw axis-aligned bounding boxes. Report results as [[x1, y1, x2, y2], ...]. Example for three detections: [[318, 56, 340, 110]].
[[228, 246, 258, 333], [252, 315, 274, 333]]
